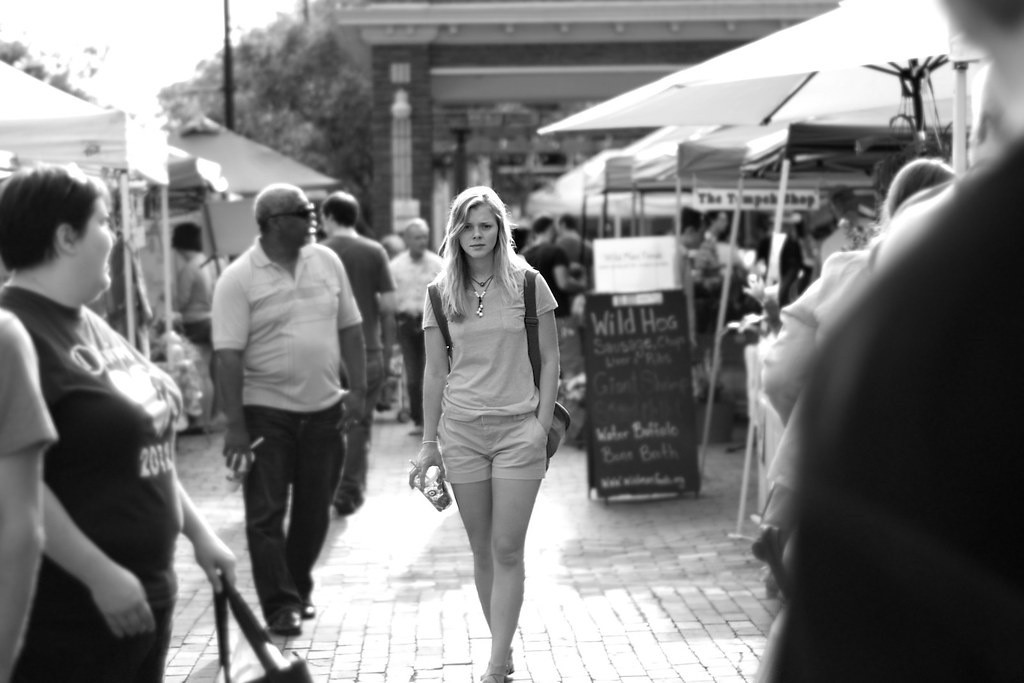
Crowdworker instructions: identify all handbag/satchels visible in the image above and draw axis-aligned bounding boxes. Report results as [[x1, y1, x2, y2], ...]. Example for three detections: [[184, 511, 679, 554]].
[[212, 574, 312, 683]]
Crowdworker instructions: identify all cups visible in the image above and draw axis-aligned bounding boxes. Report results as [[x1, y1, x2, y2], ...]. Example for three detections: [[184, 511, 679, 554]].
[[413, 466, 453, 512], [220, 451, 255, 494]]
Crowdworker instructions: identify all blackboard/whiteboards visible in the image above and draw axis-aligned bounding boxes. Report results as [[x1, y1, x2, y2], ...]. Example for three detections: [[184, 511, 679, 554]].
[[585, 289, 697, 496]]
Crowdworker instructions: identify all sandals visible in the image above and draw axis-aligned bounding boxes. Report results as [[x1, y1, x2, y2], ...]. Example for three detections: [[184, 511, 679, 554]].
[[507, 646, 515, 673], [478, 663, 515, 683]]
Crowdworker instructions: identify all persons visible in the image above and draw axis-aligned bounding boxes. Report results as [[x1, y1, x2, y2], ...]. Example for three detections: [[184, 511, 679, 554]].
[[743, 0, 1024, 683], [0, 159, 962, 683]]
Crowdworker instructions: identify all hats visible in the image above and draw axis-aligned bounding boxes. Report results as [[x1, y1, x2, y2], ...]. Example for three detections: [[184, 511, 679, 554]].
[[170, 223, 204, 251]]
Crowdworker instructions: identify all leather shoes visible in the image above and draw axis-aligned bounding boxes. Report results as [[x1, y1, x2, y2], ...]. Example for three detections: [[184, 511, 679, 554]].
[[270, 609, 301, 635], [298, 602, 315, 619]]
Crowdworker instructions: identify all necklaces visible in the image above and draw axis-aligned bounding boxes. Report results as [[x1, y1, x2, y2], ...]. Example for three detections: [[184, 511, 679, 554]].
[[469, 274, 494, 318], [471, 276, 492, 286]]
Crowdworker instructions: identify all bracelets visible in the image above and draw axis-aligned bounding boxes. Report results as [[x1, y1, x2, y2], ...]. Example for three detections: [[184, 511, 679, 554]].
[[422, 440, 438, 445]]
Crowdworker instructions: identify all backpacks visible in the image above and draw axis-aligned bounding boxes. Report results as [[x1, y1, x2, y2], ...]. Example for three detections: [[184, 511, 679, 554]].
[[427, 268, 570, 471]]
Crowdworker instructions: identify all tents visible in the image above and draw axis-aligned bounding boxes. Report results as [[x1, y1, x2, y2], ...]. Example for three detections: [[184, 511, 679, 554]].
[[0, 54, 937, 530]]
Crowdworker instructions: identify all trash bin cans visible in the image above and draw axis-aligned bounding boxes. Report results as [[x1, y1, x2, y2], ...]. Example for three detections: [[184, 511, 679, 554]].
[[397, 304, 426, 426]]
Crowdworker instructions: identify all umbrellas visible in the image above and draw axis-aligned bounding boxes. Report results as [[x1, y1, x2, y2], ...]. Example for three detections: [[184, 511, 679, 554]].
[[537, 0, 994, 148]]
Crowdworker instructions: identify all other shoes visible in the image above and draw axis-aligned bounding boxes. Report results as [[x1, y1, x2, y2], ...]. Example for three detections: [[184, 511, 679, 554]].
[[209, 414, 226, 432], [334, 487, 355, 515]]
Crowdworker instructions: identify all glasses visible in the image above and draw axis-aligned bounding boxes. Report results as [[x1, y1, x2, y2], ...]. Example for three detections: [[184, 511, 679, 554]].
[[268, 203, 316, 219]]
